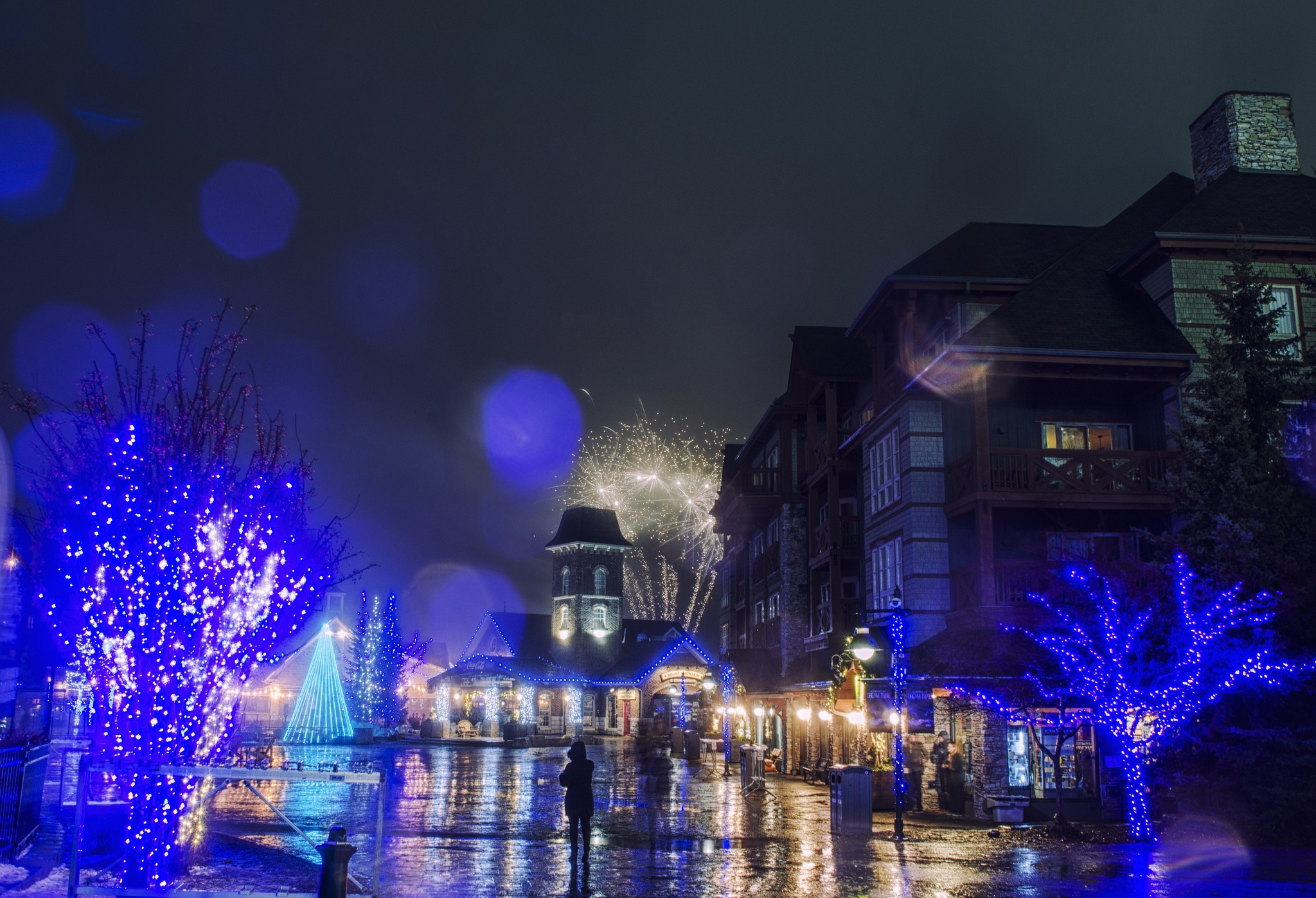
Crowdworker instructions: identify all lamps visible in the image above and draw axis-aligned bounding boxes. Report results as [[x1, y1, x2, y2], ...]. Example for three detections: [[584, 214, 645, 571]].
[[669, 678, 677, 694], [843, 620, 884, 660], [701, 667, 714, 689]]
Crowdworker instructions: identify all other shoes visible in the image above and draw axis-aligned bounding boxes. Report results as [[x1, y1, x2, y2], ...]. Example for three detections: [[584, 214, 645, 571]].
[[581, 852, 589, 862], [569, 853, 578, 859]]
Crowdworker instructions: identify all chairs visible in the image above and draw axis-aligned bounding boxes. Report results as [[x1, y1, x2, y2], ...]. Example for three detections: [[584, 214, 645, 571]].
[[457, 720, 479, 738], [765, 748, 781, 772]]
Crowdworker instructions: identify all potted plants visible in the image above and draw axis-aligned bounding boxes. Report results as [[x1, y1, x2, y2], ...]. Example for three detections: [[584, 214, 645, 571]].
[[790, 762, 797, 775]]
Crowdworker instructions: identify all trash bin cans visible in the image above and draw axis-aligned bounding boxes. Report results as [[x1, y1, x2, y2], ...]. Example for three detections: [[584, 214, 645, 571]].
[[684, 730, 700, 759], [739, 744, 769, 791], [829, 763, 873, 836], [670, 726, 684, 753]]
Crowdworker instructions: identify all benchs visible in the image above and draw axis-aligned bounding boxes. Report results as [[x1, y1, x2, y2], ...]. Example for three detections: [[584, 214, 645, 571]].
[[802, 757, 829, 785]]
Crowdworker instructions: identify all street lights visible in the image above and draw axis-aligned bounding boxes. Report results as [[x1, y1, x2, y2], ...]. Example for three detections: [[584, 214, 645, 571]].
[[702, 663, 733, 777], [845, 585, 915, 840], [669, 672, 687, 728]]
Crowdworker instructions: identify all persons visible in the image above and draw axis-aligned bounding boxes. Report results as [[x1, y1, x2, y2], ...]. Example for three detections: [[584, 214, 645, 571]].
[[559, 741, 595, 864], [636, 714, 673, 775], [941, 741, 965, 817], [930, 729, 951, 814], [902, 731, 929, 813]]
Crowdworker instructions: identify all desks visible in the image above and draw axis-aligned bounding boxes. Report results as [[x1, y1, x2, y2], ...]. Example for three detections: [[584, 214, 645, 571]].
[[693, 738, 728, 780]]
